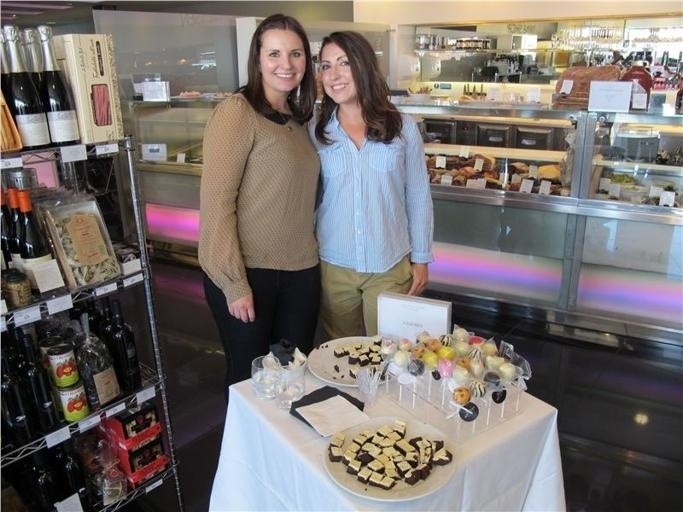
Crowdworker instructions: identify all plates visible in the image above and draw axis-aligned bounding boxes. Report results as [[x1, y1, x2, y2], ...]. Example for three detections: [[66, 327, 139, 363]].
[[324, 416, 459, 503], [171, 93, 231, 102], [307, 336, 394, 388]]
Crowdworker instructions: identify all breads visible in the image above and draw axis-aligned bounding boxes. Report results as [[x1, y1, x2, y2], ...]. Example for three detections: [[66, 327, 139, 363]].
[[557, 65, 621, 99], [427, 151, 571, 197], [459, 96, 472, 105]]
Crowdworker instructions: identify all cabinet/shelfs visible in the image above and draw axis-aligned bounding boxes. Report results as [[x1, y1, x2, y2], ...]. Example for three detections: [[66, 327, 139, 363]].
[[0, 135, 185, 510], [123, 92, 682, 361]]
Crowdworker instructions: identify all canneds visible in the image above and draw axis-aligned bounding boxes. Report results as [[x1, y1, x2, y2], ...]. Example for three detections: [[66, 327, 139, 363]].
[[40, 336, 89, 422], [456, 39, 491, 49], [1, 268, 32, 308]]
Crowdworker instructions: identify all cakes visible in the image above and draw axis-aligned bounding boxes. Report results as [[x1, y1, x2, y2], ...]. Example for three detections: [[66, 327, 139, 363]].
[[328, 420, 452, 490], [605, 168, 679, 208], [333, 337, 387, 380]]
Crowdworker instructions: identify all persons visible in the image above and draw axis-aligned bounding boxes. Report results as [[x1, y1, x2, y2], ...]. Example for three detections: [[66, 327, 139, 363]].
[[307, 30, 435, 347], [197, 13, 320, 404]]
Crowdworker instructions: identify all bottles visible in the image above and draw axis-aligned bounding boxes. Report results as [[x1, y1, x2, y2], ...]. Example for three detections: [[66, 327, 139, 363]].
[[2, 443, 88, 512], [0, 24, 82, 149], [0, 164, 57, 306], [1, 281, 146, 460]]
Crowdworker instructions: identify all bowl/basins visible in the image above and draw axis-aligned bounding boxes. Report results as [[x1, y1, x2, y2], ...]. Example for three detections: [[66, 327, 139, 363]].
[[409, 94, 430, 102]]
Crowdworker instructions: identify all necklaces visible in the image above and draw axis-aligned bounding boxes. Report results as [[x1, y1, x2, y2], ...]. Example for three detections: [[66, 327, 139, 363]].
[[278, 106, 295, 132]]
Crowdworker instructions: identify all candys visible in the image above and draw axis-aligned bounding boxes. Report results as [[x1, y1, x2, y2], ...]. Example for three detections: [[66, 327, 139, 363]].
[[378, 328, 518, 420]]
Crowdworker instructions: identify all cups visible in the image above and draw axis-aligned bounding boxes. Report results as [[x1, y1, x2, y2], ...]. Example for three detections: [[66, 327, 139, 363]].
[[250, 355, 304, 410]]
